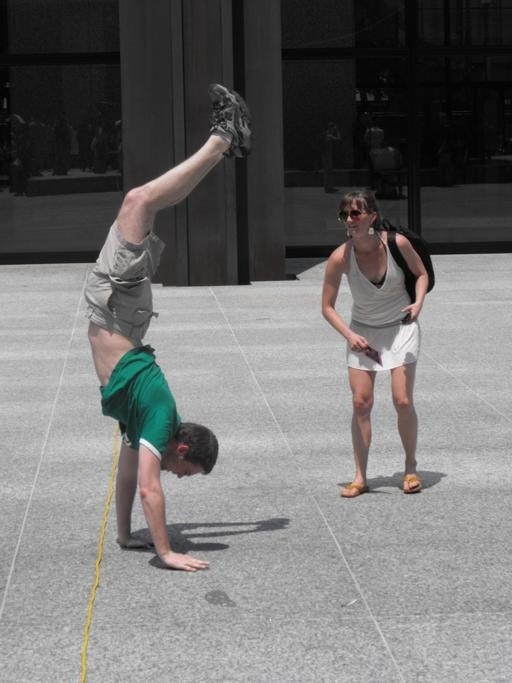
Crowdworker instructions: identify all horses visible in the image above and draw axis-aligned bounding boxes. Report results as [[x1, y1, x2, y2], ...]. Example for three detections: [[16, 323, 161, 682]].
[[402, 313, 410, 324]]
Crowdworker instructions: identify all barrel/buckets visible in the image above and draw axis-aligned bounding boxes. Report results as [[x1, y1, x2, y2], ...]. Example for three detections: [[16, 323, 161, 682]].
[[340, 482, 371, 497], [402, 474, 423, 494]]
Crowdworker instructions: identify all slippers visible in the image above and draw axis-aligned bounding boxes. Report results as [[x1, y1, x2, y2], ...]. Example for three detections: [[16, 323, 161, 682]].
[[379, 217, 437, 303]]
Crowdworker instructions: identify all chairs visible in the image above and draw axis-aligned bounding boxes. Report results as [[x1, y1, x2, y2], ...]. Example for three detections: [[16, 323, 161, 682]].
[[206, 81, 256, 160]]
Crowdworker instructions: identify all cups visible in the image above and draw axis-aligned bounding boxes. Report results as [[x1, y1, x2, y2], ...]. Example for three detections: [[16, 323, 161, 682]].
[[336, 208, 370, 222]]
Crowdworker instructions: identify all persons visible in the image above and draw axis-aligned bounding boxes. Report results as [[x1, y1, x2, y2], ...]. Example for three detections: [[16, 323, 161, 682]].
[[321, 187, 432, 498], [86, 78, 254, 574], [319, 122, 342, 193], [1, 110, 124, 195]]
